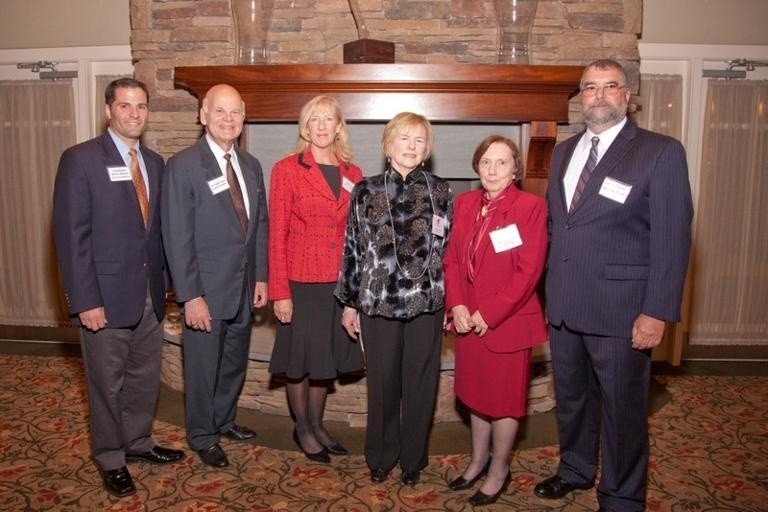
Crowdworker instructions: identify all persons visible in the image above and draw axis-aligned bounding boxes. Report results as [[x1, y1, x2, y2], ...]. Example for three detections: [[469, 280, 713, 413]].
[[267, 93, 366, 464], [53, 79, 184, 499], [161, 84, 267, 468], [334, 112, 452, 486], [444, 135, 549, 507], [533, 61, 694, 512]]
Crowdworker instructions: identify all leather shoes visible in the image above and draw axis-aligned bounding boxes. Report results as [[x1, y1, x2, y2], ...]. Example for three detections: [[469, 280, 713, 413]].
[[371, 468, 387, 483], [221, 424, 257, 442], [533, 475, 594, 500], [402, 469, 419, 487], [197, 442, 229, 468], [125, 444, 184, 465], [103, 466, 137, 498]]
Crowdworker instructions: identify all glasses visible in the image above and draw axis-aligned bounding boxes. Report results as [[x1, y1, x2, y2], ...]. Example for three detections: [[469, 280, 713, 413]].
[[580, 82, 626, 95]]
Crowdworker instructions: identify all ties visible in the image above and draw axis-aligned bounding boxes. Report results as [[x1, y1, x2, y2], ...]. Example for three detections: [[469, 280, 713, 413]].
[[223, 153, 249, 237], [569, 136, 600, 215], [128, 147, 149, 230]]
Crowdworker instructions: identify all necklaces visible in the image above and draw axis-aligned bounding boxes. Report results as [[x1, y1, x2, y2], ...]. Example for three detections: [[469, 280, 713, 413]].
[[384, 169, 437, 281], [481, 193, 505, 217]]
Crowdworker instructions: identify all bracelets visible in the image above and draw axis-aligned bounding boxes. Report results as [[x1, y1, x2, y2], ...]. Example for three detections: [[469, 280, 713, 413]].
[[341, 309, 357, 315]]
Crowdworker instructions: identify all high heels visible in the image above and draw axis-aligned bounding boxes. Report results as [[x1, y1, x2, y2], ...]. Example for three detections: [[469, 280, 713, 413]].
[[292, 425, 331, 465], [329, 443, 350, 456], [449, 455, 492, 490], [469, 469, 512, 506]]
